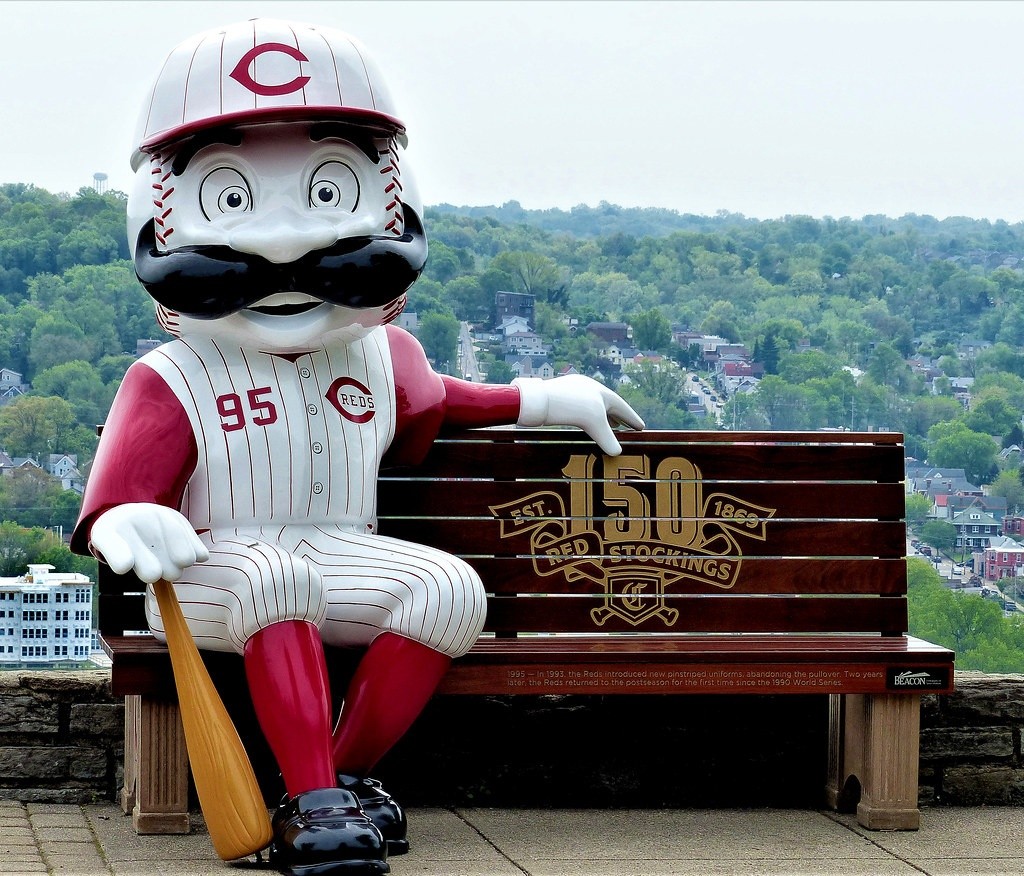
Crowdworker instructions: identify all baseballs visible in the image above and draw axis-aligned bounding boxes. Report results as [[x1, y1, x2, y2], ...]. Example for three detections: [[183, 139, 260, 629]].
[[151, 579, 278, 860]]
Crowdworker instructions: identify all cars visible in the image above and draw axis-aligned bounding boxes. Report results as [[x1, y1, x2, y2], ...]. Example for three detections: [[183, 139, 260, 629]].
[[1005, 601, 1017, 611], [692, 376, 724, 408], [457, 336, 462, 345], [973, 577, 1001, 599], [911, 539, 942, 563], [458, 351, 464, 358], [952, 567, 962, 576], [956, 559, 972, 568]]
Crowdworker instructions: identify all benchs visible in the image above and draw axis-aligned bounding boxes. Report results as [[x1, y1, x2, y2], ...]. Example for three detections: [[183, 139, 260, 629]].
[[93, 423, 957, 830]]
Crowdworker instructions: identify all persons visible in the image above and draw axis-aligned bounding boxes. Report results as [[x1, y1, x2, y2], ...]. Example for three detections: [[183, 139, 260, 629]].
[[70, 18, 646, 876]]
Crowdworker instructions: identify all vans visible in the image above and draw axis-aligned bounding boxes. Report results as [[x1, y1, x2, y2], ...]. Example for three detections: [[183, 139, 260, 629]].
[[465, 373, 472, 381]]
